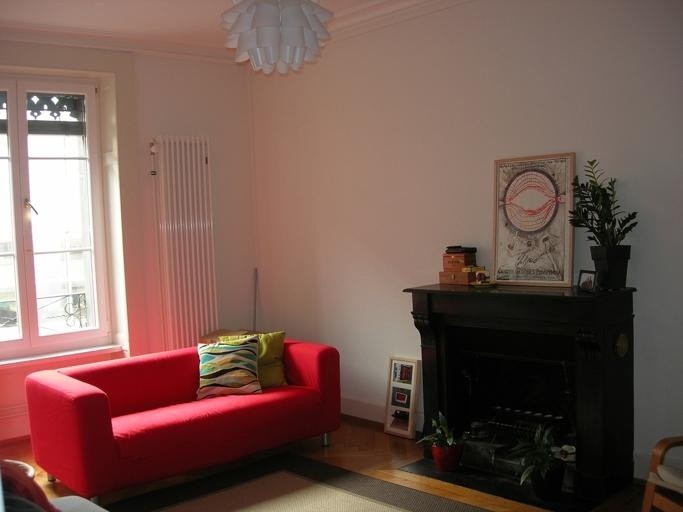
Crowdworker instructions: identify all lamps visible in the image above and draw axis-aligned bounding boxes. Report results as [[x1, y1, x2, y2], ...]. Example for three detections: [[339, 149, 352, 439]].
[[218, 0, 335, 75]]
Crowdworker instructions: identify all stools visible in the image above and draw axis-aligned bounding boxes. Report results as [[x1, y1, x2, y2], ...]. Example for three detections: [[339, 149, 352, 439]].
[[640, 436, 682, 511], [53, 495, 110, 512]]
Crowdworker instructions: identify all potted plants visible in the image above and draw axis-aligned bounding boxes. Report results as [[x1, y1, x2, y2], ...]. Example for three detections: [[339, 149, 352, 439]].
[[416, 410, 464, 473], [567, 159, 639, 290], [506, 423, 567, 501]]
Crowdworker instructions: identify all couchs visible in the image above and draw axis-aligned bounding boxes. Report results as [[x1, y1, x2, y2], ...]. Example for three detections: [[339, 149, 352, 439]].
[[26, 339, 342, 505]]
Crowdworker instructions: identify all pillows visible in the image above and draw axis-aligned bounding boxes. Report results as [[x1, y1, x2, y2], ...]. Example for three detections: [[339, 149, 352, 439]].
[[196, 335, 262, 400], [217, 329, 288, 389]]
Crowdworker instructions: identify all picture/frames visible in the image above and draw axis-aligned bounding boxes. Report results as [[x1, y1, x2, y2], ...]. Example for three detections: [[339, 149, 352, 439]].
[[490, 152, 576, 288], [383, 355, 423, 440], [576, 269, 598, 293]]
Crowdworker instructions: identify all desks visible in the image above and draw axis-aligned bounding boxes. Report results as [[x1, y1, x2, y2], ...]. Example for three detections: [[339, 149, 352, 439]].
[[403, 283, 635, 507]]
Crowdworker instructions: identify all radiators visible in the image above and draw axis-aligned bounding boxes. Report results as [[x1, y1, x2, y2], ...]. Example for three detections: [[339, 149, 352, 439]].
[[151, 133, 221, 351]]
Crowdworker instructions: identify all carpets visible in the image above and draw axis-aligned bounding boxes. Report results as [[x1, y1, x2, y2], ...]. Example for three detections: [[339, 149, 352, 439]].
[[103, 453, 496, 511]]
